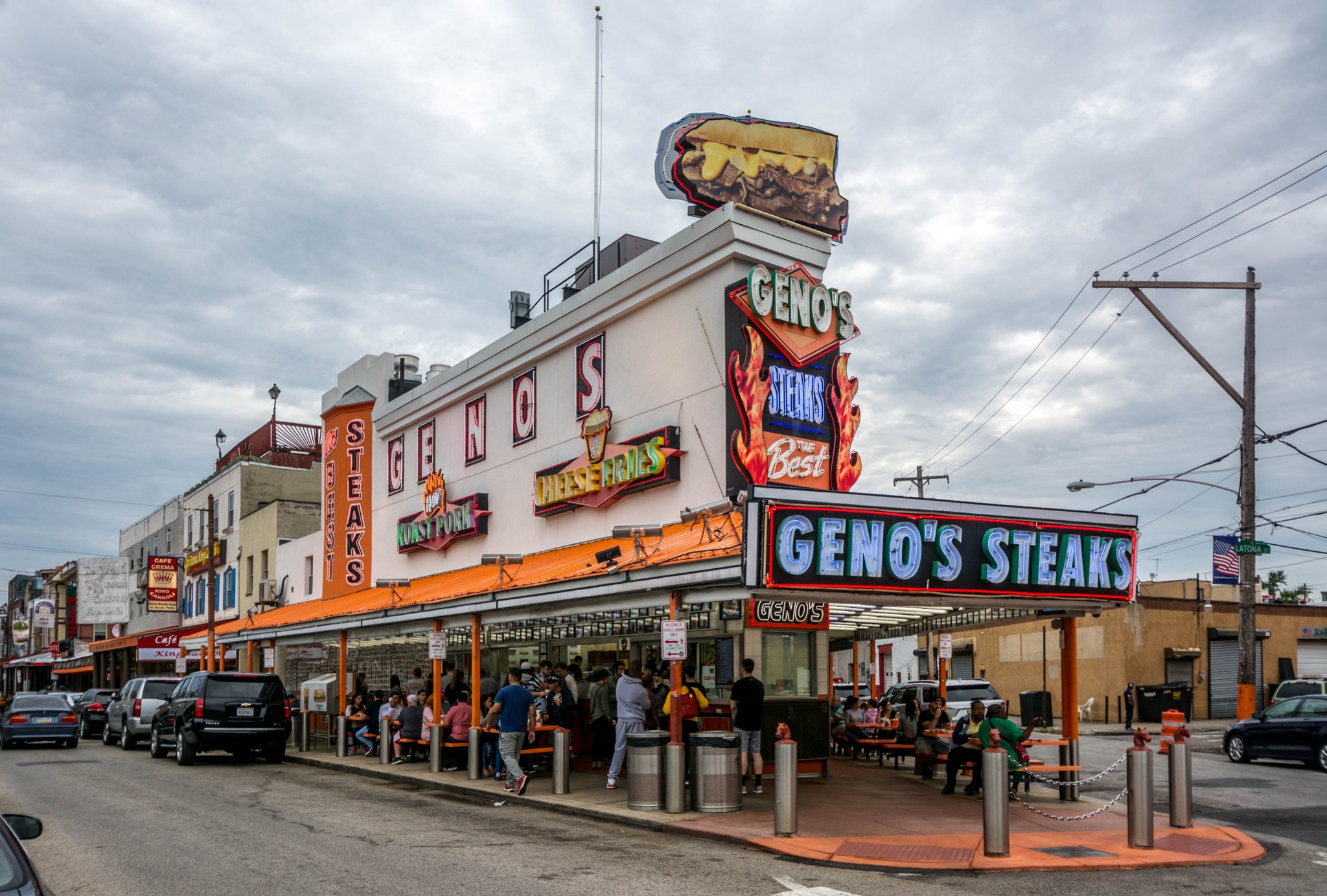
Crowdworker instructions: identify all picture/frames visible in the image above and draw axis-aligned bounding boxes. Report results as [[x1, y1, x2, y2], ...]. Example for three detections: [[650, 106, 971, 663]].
[[441, 599, 742, 646]]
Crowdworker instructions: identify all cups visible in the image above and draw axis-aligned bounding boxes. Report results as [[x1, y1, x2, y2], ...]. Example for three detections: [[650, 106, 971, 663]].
[[535, 717, 540, 726], [304, 696, 309, 710], [555, 693, 562, 703], [541, 719, 542, 726], [536, 710, 541, 717], [441, 715, 444, 724], [879, 718, 885, 727], [398, 713, 400, 716], [545, 689, 551, 699], [553, 697, 557, 700]]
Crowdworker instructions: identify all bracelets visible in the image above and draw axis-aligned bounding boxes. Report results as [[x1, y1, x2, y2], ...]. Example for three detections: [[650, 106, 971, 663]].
[[529, 732, 534, 733]]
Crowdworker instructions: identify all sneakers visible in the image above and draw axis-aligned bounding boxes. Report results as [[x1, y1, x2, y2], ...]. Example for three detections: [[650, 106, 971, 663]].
[[607, 778, 616, 788], [597, 762, 608, 769], [753, 785, 763, 793], [942, 782, 1016, 800], [391, 757, 402, 764], [376, 746, 380, 757], [360, 744, 369, 754], [592, 762, 597, 769], [484, 765, 539, 796], [407, 754, 411, 757], [349, 747, 355, 755], [742, 786, 747, 794], [365, 748, 373, 757]]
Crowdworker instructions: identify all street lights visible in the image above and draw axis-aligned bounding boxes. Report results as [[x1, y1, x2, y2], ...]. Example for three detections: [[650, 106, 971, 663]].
[[1065, 476, 1256, 722]]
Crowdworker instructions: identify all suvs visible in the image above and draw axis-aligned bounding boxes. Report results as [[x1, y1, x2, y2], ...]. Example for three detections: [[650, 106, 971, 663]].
[[1269, 674, 1327, 707], [150, 671, 292, 766], [833, 681, 878, 706], [879, 678, 1006, 726], [102, 674, 184, 751]]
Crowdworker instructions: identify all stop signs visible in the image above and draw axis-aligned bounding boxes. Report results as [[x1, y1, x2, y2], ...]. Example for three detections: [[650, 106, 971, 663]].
[[48, 641, 59, 655]]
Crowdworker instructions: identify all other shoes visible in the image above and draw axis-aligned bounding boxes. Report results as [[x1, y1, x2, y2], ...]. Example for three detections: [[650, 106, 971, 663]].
[[419, 755, 424, 759], [571, 753, 575, 758], [448, 766, 457, 770]]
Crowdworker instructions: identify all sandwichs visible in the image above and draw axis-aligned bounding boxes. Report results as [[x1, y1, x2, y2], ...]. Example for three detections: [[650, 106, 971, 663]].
[[676, 120, 850, 236]]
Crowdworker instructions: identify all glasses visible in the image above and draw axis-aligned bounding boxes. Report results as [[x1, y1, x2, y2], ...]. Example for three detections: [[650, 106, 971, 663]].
[[542, 668, 552, 671], [974, 708, 985, 711]]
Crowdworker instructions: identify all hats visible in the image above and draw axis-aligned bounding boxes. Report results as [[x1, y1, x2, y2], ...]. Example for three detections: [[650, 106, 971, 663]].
[[440, 690, 447, 699], [521, 662, 531, 669], [929, 697, 942, 702], [510, 667, 522, 677], [544, 675, 560, 683], [406, 694, 418, 703]]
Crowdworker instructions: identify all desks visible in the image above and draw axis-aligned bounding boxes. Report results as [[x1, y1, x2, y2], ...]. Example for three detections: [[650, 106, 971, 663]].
[[967, 737, 1068, 794], [922, 732, 953, 779], [482, 725, 560, 732], [348, 716, 369, 720], [848, 722, 899, 765], [426, 723, 452, 728]]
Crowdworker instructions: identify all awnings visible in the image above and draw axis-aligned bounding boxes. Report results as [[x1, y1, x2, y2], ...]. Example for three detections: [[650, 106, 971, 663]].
[[89, 628, 179, 651]]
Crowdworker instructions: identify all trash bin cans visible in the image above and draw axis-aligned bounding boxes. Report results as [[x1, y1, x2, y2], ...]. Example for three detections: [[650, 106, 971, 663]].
[[1136, 680, 1192, 723], [689, 729, 741, 813], [625, 730, 669, 810], [1020, 690, 1054, 728]]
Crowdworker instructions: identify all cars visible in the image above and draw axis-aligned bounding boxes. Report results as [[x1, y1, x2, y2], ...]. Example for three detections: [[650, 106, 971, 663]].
[[72, 688, 121, 739], [0, 683, 84, 750], [1222, 694, 1327, 773]]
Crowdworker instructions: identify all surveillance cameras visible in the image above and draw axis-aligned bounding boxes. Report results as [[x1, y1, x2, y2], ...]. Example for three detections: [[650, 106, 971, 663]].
[[1090, 608, 1103, 618]]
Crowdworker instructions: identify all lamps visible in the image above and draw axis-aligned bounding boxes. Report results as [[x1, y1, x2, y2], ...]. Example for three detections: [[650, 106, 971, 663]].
[[247, 606, 259, 625], [611, 524, 663, 561], [1193, 599, 1213, 613], [375, 578, 410, 604], [595, 545, 622, 566], [480, 554, 524, 585], [680, 496, 732, 543]]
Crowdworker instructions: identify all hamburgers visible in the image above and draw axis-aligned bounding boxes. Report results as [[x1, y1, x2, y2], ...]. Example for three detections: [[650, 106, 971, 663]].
[[314, 689, 326, 702]]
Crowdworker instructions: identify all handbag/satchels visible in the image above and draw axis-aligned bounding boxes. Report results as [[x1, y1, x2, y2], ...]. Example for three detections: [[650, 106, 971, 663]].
[[588, 722, 592, 733], [1012, 742, 1029, 763]]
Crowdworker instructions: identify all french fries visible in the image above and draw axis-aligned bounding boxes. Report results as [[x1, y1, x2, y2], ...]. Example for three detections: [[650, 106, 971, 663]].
[[304, 686, 310, 696]]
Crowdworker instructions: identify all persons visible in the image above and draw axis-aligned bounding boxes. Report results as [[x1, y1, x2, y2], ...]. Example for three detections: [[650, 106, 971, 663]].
[[340, 653, 767, 796], [830, 696, 1045, 799], [1123, 682, 1136, 731]]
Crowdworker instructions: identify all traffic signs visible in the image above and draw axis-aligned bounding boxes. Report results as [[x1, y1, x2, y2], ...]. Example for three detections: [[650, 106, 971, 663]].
[[661, 619, 687, 660]]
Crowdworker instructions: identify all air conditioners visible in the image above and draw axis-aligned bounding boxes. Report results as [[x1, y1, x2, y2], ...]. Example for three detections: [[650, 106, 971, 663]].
[[137, 557, 143, 565], [258, 580, 277, 602]]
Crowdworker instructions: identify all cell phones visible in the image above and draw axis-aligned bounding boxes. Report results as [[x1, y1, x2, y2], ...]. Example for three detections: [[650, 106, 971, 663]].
[[389, 697, 392, 702]]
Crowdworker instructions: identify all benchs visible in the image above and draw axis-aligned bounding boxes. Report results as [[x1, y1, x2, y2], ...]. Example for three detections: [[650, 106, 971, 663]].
[[346, 731, 573, 754], [837, 734, 1082, 771]]
